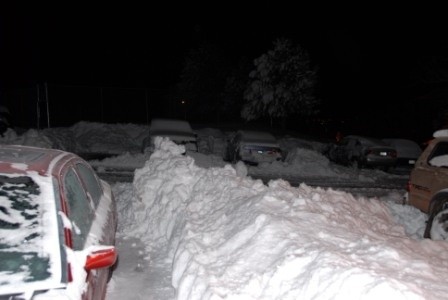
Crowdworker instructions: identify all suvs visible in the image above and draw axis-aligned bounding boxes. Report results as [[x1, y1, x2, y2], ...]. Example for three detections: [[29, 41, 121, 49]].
[[406, 129, 448, 239], [141, 120, 200, 155]]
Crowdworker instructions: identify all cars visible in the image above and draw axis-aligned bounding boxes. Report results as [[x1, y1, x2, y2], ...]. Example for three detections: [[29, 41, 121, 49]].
[[222, 131, 284, 167], [327, 136, 397, 173], [0, 144, 119, 300], [383, 138, 424, 174], [70, 122, 121, 159]]
